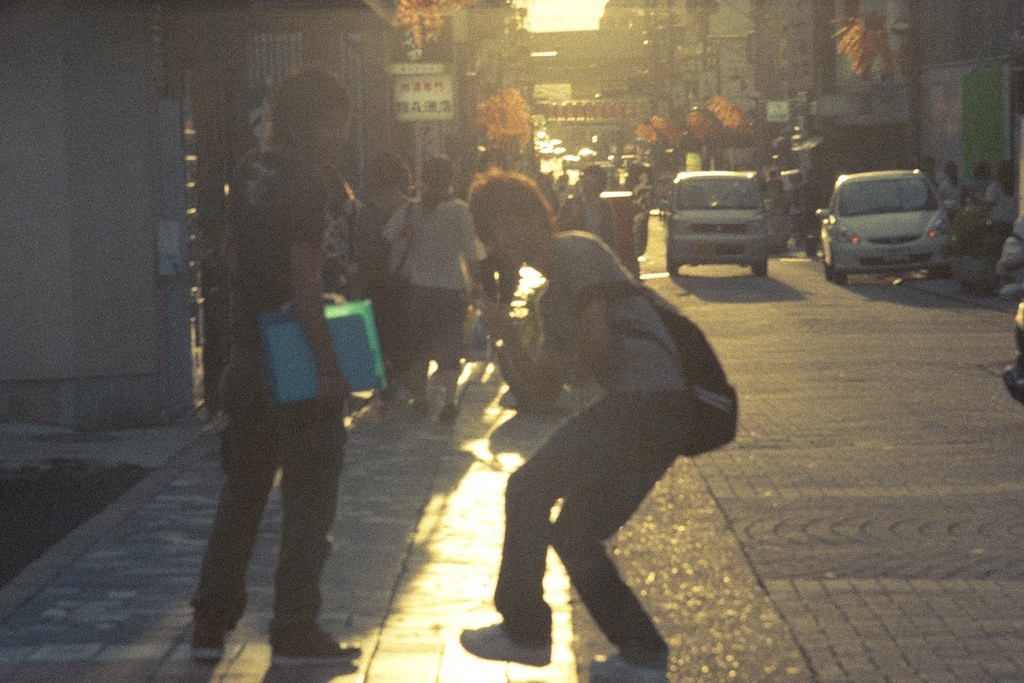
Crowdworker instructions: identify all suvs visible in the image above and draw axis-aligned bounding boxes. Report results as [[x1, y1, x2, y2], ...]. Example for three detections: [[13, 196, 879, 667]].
[[665, 170, 777, 277]]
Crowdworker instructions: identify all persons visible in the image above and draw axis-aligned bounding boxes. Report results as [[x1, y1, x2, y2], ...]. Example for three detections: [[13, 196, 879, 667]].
[[459, 168, 694, 683], [322, 153, 1024, 425], [189, 63, 361, 668]]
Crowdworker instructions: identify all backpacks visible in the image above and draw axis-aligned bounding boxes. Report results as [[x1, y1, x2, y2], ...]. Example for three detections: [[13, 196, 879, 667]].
[[580, 281, 739, 458]]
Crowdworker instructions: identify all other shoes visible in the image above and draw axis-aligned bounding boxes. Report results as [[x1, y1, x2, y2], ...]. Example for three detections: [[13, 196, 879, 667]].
[[461, 622, 552, 667], [414, 400, 430, 418], [193, 618, 226, 662], [440, 400, 460, 424], [270, 630, 362, 666], [591, 654, 667, 683]]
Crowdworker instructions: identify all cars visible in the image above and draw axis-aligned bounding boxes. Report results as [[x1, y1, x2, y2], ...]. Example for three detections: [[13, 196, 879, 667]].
[[815, 169, 959, 286]]
